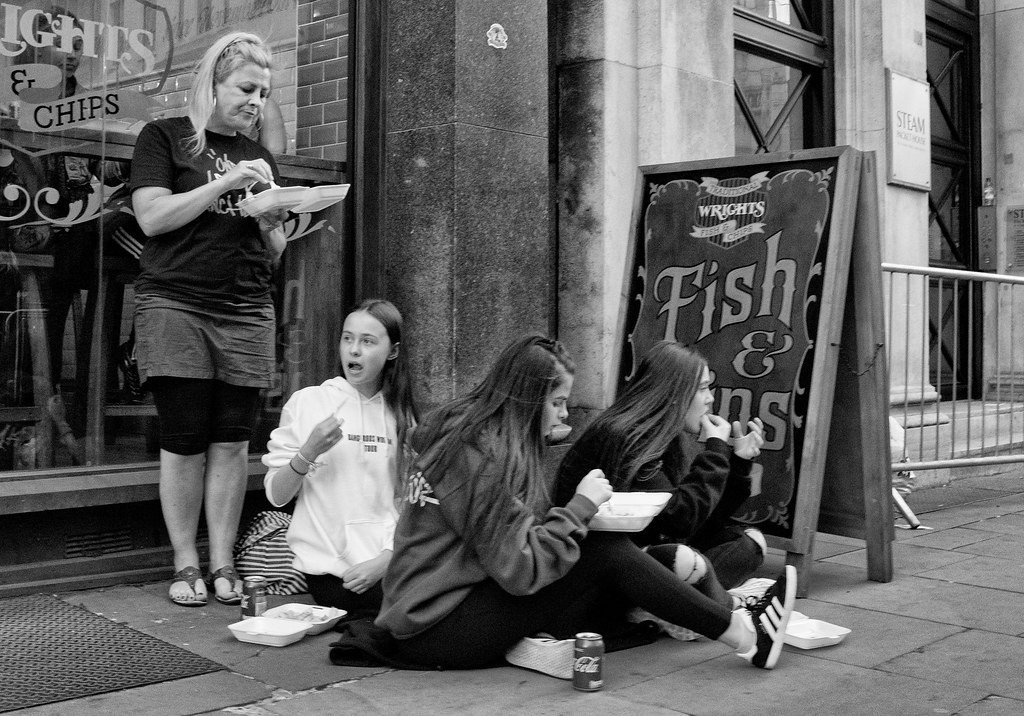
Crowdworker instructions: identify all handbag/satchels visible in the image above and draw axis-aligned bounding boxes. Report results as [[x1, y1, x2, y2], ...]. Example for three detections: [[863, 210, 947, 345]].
[[233, 511, 309, 597]]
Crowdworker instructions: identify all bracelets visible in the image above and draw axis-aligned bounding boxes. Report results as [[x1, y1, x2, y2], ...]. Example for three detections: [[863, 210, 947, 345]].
[[297, 450, 314, 465], [289, 460, 309, 475]]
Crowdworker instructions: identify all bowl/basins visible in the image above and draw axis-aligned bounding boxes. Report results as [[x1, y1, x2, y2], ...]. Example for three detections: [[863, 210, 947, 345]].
[[783, 611, 851, 650], [587, 491, 673, 532], [229, 603, 348, 647], [235, 184, 350, 218]]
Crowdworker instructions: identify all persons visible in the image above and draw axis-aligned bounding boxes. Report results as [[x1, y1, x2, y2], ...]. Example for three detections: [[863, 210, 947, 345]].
[[555, 341, 768, 640], [130, 32, 289, 607], [374, 334, 798, 680], [261, 297, 423, 618], [0, 6, 128, 436]]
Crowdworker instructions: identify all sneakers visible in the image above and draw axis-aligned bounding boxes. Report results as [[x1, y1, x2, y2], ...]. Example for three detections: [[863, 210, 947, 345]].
[[743, 563, 798, 670], [506, 632, 577, 680]]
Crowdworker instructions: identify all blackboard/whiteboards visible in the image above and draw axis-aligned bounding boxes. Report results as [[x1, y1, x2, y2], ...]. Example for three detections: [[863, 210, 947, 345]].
[[604, 145, 863, 557]]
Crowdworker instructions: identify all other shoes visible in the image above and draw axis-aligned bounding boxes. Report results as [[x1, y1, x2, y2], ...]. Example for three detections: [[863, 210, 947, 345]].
[[630, 607, 705, 641], [730, 577, 776, 603]]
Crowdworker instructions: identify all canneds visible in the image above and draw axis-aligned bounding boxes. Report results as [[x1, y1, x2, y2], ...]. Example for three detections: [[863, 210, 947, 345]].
[[241, 575, 267, 619], [572, 632, 605, 692]]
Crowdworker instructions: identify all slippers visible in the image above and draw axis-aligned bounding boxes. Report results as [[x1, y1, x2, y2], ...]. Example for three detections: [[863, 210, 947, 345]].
[[205, 566, 243, 604], [170, 566, 207, 605]]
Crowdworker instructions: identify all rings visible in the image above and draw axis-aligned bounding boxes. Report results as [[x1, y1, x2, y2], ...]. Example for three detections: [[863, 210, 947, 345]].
[[247, 164, 253, 170]]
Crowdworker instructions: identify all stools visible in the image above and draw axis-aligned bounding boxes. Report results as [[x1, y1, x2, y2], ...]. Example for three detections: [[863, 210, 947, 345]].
[[0, 250, 57, 472]]
